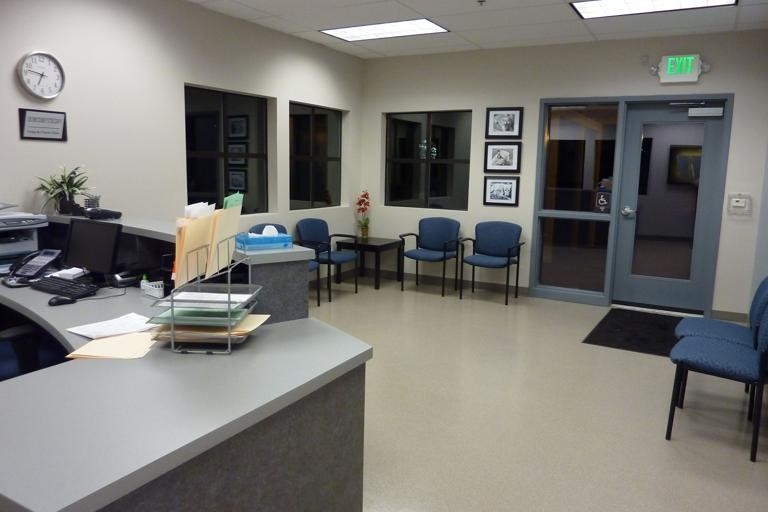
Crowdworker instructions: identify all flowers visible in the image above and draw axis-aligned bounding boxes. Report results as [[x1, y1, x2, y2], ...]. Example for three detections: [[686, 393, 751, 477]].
[[353, 191, 370, 226]]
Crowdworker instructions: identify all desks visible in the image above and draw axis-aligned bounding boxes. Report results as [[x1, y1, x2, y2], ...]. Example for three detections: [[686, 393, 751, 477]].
[[336, 237, 402, 290], [0, 212, 373, 512]]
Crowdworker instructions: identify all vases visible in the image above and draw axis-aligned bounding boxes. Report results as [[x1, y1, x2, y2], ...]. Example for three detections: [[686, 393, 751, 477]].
[[361, 226, 368, 240]]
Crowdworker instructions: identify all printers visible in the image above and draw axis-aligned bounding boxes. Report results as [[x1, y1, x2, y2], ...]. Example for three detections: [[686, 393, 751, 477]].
[[0, 210, 49, 276]]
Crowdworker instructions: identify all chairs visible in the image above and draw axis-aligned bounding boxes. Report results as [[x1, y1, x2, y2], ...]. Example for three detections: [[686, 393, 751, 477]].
[[666, 304, 768, 461], [399, 217, 460, 297], [674, 276, 768, 408], [459, 222, 525, 305], [297, 218, 358, 302], [250, 223, 322, 306]]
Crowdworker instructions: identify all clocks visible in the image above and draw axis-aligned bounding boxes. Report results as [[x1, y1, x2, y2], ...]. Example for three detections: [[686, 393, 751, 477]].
[[18, 51, 66, 99]]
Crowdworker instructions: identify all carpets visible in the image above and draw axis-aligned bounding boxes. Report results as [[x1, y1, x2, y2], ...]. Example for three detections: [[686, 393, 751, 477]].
[[584, 309, 683, 357]]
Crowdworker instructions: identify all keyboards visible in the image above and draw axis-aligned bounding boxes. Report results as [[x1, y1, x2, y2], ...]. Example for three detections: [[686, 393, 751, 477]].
[[31, 275, 96, 299]]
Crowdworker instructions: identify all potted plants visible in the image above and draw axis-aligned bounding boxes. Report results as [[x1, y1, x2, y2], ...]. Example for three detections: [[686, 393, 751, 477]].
[[34, 166, 97, 215]]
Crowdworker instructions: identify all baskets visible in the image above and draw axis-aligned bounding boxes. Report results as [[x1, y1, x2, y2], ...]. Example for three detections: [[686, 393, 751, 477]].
[[145, 281, 165, 298]]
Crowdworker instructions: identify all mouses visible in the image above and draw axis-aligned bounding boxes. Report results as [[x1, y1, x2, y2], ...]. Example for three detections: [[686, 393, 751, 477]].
[[48, 296, 76, 306]]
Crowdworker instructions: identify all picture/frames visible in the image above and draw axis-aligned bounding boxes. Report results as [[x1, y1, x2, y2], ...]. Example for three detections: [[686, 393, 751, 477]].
[[225, 115, 249, 140], [485, 142, 521, 173], [226, 142, 248, 167], [486, 107, 523, 139], [484, 176, 519, 207], [227, 169, 247, 193]]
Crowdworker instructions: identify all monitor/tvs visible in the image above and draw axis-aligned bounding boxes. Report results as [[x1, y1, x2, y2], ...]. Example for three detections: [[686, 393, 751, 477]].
[[62, 217, 123, 288]]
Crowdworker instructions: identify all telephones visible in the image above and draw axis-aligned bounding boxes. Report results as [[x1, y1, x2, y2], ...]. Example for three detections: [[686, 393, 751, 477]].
[[9, 248, 63, 279]]
